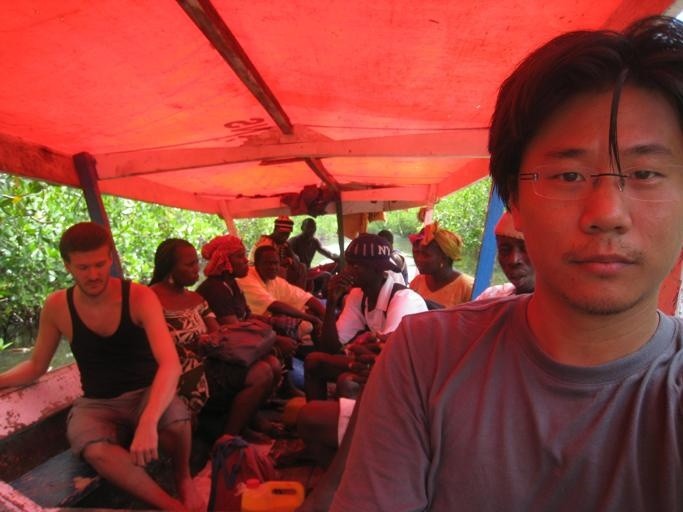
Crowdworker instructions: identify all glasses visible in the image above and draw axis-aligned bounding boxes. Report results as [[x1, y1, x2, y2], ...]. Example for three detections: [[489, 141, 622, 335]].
[[511, 160, 682, 204]]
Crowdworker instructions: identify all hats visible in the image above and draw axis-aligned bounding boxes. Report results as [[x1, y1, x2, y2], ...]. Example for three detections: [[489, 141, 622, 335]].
[[275, 214, 294, 232], [346, 233, 402, 273]]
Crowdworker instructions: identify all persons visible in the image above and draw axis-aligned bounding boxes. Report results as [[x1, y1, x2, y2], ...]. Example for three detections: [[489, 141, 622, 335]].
[[300, 10, 681, 510], [0, 221, 211, 512], [467, 210, 538, 302], [146, 213, 475, 468]]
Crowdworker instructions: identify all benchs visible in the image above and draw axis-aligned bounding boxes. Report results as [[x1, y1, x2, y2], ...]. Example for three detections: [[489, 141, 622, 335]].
[[10, 428, 166, 511]]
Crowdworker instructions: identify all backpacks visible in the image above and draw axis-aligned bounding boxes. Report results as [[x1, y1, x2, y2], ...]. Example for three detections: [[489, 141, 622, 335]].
[[211, 433, 276, 510]]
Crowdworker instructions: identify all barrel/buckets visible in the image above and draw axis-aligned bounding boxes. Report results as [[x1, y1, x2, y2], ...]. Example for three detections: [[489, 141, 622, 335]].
[[240, 478, 305, 512]]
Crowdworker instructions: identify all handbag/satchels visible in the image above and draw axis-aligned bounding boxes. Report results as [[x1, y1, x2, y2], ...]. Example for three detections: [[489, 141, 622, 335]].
[[203, 318, 277, 368]]
